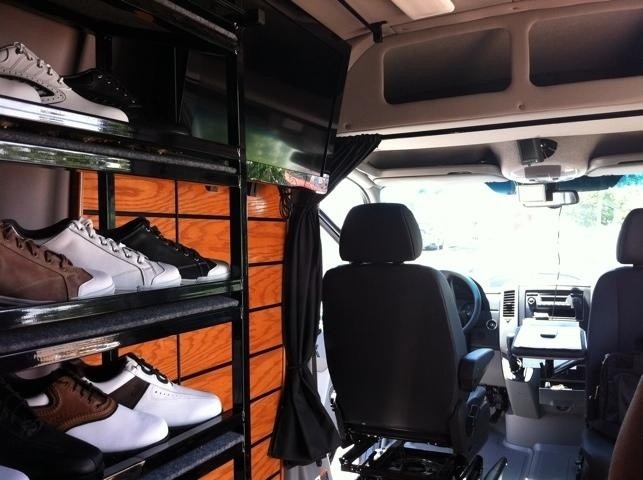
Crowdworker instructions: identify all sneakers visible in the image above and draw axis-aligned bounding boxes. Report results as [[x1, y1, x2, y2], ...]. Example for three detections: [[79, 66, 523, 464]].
[[0, 352, 221, 479], [0, 40, 194, 137], [0, 213, 230, 303]]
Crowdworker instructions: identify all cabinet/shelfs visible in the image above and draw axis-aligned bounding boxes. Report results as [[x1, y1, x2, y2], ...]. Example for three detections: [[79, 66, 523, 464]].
[[0, 0, 251, 480]]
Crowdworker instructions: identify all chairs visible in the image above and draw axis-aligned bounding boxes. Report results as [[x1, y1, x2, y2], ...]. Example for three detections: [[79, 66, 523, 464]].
[[579, 208, 643, 477], [323, 203, 495, 467]]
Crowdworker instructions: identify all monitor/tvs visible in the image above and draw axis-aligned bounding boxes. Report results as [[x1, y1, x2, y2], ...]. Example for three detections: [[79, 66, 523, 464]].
[[176, 0, 352, 195]]
